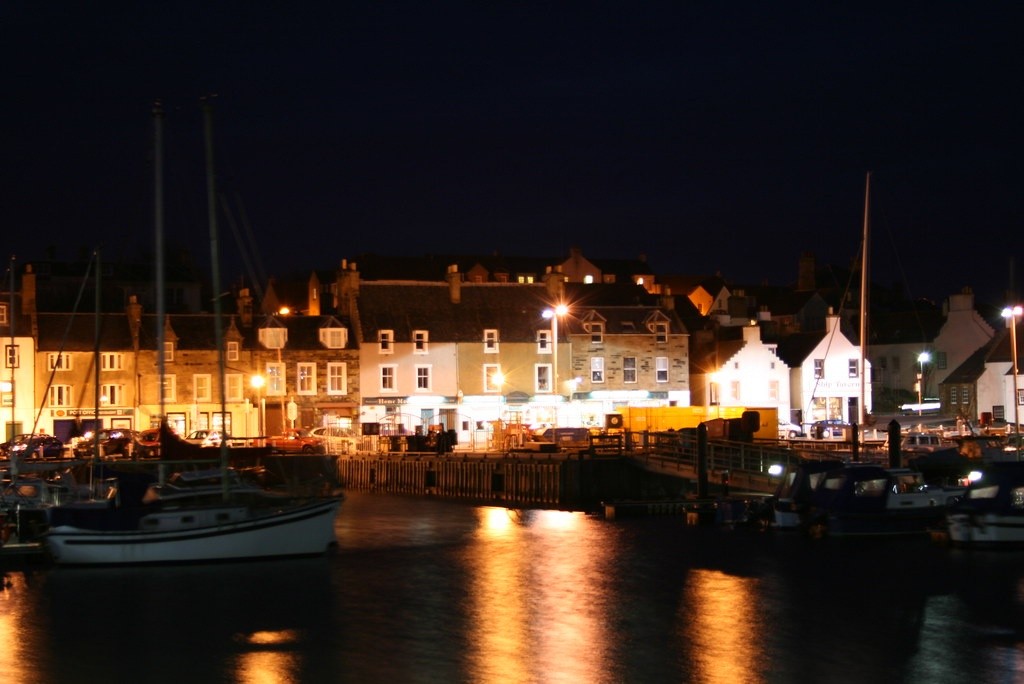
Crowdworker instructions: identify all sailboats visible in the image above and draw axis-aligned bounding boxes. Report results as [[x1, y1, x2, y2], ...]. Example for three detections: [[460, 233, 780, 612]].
[[0, 92, 345, 568]]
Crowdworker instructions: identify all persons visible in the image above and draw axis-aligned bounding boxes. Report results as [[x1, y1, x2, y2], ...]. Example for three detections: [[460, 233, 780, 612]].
[[956, 415, 969, 431], [435, 423, 453, 453]]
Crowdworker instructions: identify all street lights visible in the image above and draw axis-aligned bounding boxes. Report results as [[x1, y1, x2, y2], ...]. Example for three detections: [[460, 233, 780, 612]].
[[917, 352, 930, 417], [542, 304, 569, 395], [1001, 306, 1023, 434]]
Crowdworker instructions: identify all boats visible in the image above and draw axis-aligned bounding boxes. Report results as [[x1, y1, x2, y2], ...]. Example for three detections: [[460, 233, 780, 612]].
[[756, 459, 970, 539], [946, 461, 1024, 551]]
